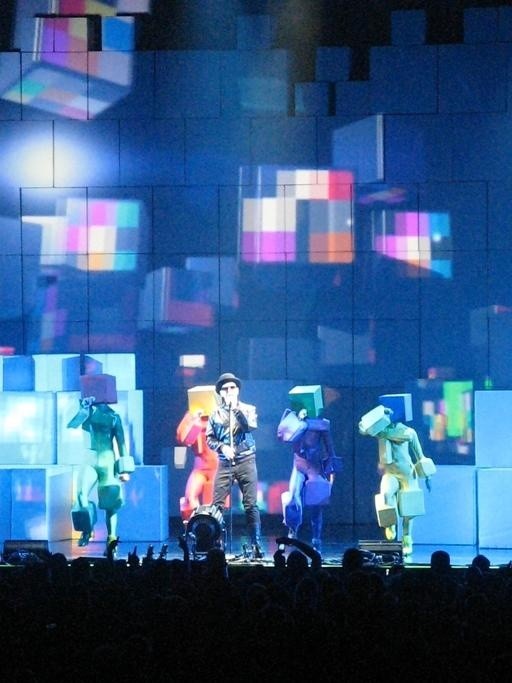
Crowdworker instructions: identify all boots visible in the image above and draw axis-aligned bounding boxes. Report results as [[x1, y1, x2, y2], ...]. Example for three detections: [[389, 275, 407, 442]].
[[385, 525, 396, 539], [103, 536, 117, 555], [248, 522, 265, 557], [403, 536, 412, 555], [78, 531, 91, 546], [312, 538, 322, 558]]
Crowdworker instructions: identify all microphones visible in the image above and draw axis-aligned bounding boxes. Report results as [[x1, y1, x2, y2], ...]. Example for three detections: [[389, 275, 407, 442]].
[[384, 409, 393, 415], [85, 396, 96, 405]]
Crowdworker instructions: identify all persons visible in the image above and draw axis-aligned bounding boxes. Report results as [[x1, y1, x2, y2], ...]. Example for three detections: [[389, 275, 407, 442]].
[[359, 394, 432, 564], [205, 373, 265, 560], [280, 385, 337, 559], [1, 539, 511, 682], [67, 374, 130, 560], [176, 388, 221, 513]]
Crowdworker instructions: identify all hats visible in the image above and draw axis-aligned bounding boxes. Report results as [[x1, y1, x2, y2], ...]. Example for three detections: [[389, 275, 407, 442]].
[[216, 373, 242, 394]]
